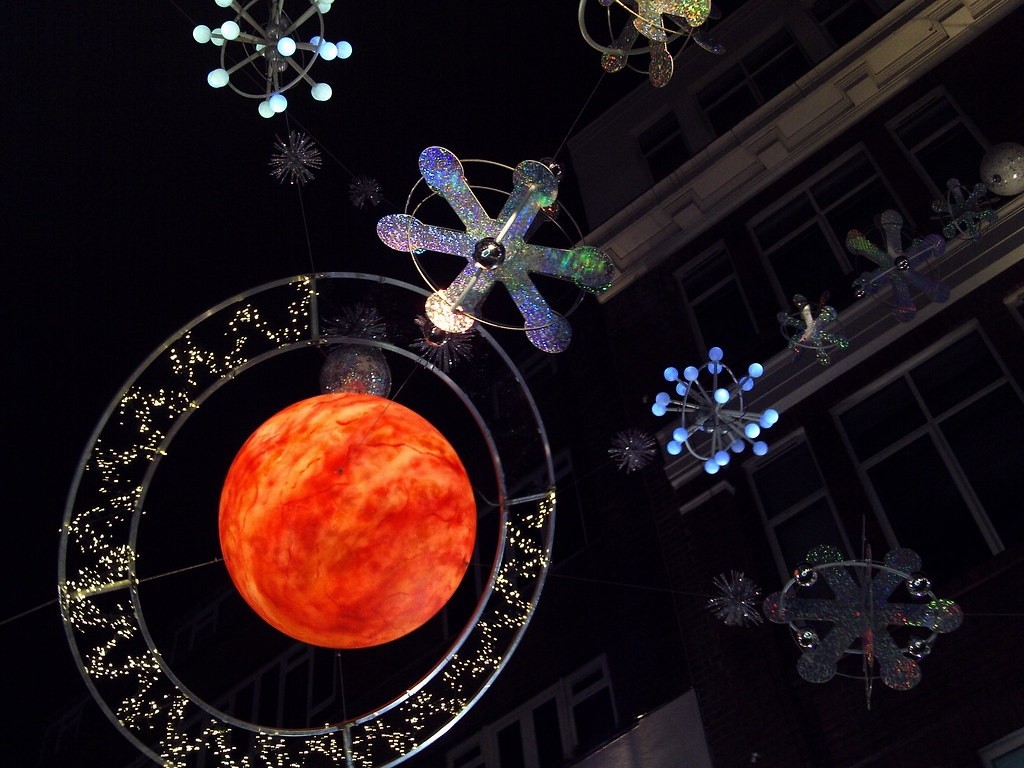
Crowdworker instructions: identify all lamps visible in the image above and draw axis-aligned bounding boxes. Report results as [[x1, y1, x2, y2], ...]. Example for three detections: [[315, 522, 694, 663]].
[[652, 347, 778, 473]]
